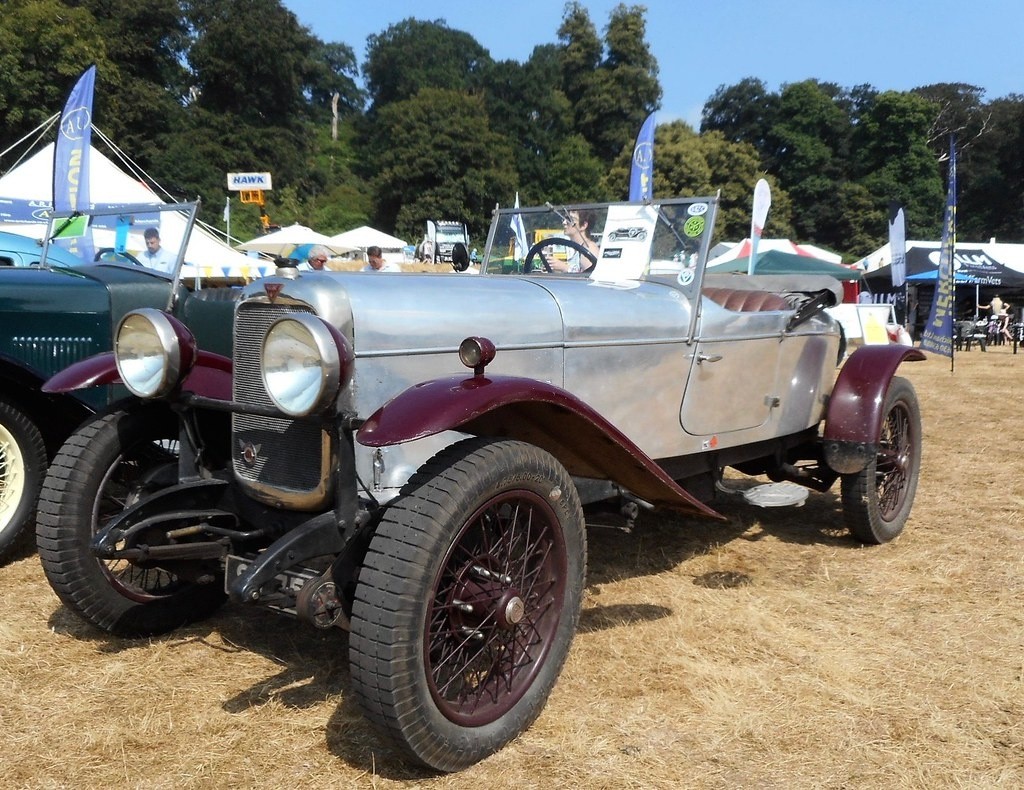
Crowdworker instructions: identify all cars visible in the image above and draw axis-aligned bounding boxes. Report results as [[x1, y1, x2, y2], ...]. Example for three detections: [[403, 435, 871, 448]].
[[0, 196, 264, 567], [39, 188, 926, 774]]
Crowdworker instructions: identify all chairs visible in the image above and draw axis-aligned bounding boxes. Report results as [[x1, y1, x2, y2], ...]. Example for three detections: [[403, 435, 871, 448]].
[[957, 321, 987, 352]]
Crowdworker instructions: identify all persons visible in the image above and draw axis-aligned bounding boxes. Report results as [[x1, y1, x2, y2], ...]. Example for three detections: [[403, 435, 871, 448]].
[[470, 248, 477, 264], [296, 246, 330, 273], [977, 293, 1012, 338], [132, 228, 184, 283], [863, 257, 869, 271], [360, 247, 401, 272], [902, 295, 912, 328], [858, 286, 874, 303], [879, 257, 884, 268], [542, 209, 600, 274]]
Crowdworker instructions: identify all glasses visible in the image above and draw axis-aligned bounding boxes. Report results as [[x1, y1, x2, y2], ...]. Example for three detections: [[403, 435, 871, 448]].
[[369, 259, 377, 264], [562, 216, 579, 222], [317, 259, 326, 264]]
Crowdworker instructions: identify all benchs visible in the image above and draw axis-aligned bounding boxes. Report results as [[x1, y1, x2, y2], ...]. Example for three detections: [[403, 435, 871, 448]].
[[699, 287, 792, 314]]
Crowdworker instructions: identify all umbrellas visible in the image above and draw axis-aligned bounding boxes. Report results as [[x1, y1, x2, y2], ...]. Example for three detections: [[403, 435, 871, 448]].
[[234, 222, 360, 258]]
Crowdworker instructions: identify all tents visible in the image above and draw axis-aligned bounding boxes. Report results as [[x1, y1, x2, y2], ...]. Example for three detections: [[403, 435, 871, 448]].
[[699, 236, 857, 303], [332, 226, 407, 253], [852, 239, 1024, 298]]
